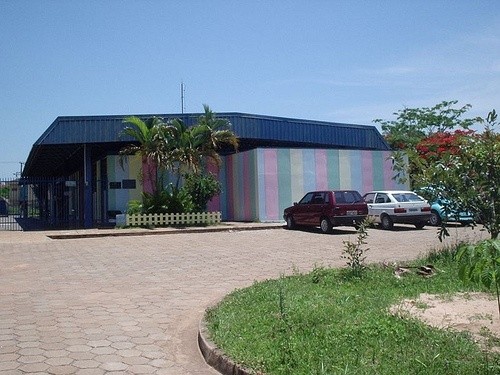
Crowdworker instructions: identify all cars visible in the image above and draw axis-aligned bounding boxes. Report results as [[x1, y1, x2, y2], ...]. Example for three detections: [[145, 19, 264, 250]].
[[415, 187, 476, 226], [362, 190, 432, 229], [283, 190, 368, 232]]
